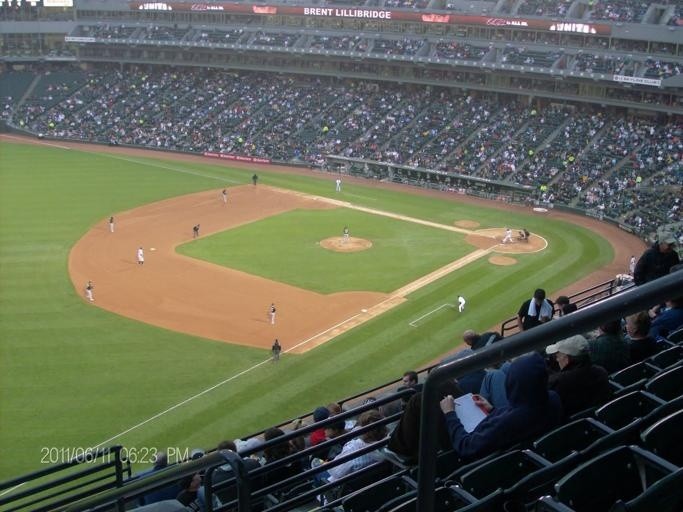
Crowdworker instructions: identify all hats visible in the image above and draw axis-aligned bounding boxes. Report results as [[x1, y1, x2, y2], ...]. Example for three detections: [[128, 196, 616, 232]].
[[533, 288, 546, 299], [155, 406, 346, 488], [545, 333, 591, 356], [659, 231, 676, 245]]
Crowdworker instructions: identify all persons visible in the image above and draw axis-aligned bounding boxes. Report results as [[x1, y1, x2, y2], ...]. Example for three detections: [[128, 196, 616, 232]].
[[272, 338, 281, 361], [388, 245, 683, 463], [140, 397, 387, 511], [87, 280, 95, 301], [1, 23, 683, 244], [270, 303, 277, 325], [138, 247, 144, 264]]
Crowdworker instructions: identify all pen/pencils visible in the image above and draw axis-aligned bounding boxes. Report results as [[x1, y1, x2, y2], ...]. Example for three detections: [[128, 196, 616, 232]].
[[443, 396, 461, 406]]
